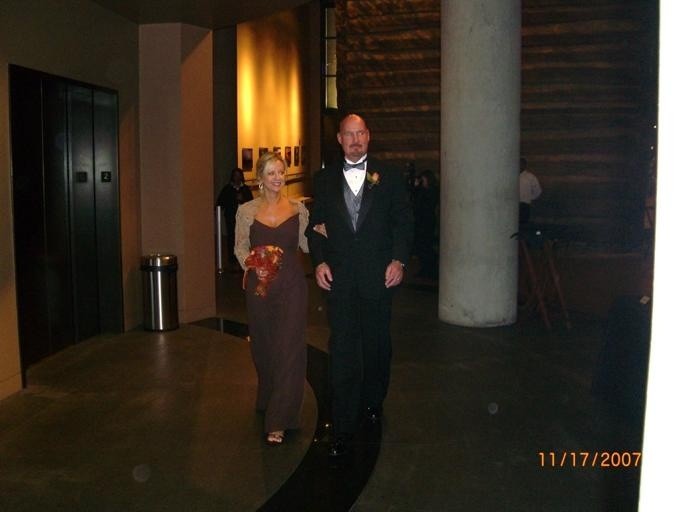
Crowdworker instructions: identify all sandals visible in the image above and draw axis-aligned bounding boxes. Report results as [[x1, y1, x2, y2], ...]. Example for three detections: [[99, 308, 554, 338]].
[[265, 430, 288, 447]]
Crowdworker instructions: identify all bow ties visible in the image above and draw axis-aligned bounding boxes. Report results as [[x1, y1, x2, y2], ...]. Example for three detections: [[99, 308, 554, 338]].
[[344, 161, 365, 171]]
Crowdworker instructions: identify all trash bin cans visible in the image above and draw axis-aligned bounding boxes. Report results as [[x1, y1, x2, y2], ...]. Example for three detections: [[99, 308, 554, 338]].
[[142, 254, 178, 331]]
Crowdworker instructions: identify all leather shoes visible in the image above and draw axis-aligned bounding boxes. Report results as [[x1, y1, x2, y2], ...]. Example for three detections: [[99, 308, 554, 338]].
[[329, 440, 348, 456], [363, 407, 379, 425]]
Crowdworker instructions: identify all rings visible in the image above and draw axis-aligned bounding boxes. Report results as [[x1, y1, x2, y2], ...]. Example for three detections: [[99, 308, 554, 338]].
[[397, 278, 400, 281]]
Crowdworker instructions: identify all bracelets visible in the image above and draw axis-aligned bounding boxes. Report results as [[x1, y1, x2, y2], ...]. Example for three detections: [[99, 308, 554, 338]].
[[392, 258, 407, 267]]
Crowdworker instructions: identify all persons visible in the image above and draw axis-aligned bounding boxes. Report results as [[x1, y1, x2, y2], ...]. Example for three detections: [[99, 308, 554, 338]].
[[233, 151, 329, 445], [413, 169, 440, 283], [304, 112, 417, 457], [215, 168, 255, 262], [520, 157, 544, 224]]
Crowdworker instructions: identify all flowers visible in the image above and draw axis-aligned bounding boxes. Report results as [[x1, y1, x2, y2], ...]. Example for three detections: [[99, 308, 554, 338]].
[[244, 245, 284, 295]]
